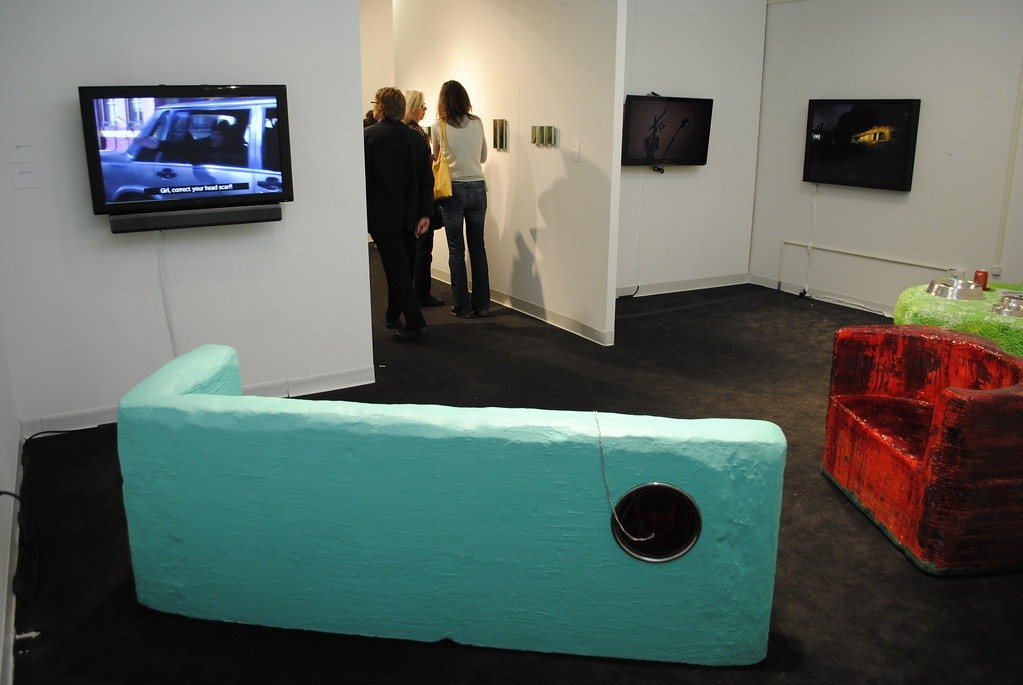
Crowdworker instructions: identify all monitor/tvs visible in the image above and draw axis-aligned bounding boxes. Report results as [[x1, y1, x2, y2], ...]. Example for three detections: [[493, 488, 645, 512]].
[[802, 99, 922, 191], [621, 95, 714, 166], [79, 84, 294, 235]]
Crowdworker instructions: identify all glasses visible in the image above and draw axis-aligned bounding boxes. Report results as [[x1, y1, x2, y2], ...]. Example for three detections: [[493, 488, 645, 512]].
[[419, 102, 425, 108], [371, 101, 379, 106]]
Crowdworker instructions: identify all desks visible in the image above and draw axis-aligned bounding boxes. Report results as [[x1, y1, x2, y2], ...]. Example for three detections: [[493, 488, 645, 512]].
[[894, 282, 1023, 358]]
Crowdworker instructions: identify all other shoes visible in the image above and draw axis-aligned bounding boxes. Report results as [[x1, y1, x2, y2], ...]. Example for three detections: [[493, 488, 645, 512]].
[[417, 295, 445, 307], [451, 304, 471, 319], [396, 324, 429, 336], [470, 305, 488, 317], [386, 320, 401, 329]]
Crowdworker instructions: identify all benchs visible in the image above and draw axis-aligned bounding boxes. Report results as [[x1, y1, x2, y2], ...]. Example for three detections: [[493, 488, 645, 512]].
[[120, 344, 787, 665]]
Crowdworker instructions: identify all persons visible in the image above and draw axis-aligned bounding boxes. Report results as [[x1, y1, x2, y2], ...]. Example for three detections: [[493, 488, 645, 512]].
[[176, 119, 236, 165], [364, 87, 445, 338], [430, 80, 490, 318]]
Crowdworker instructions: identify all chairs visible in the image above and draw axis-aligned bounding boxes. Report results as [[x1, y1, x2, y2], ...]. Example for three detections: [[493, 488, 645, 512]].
[[822, 325, 1023, 574]]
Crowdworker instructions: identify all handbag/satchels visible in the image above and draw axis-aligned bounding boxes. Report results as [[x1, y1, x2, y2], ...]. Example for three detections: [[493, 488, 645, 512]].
[[432, 117, 452, 200]]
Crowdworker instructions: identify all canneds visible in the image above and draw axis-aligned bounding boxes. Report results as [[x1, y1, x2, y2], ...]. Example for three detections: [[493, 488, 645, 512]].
[[973, 269, 988, 290]]
